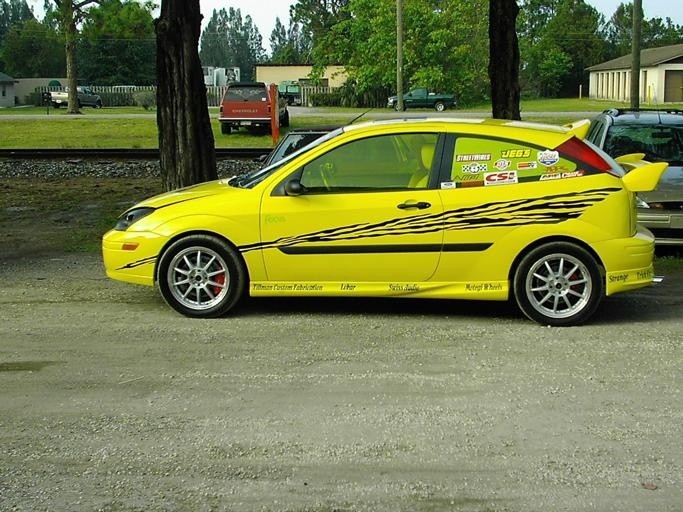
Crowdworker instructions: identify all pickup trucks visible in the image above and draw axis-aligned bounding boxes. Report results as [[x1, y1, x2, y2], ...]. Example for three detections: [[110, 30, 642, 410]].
[[387, 88, 457, 112], [50, 85, 103, 109]]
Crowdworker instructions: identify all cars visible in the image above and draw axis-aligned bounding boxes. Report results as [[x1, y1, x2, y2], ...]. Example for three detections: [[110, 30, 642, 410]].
[[584, 103, 683, 261], [217, 81, 289, 134], [100, 95, 672, 328]]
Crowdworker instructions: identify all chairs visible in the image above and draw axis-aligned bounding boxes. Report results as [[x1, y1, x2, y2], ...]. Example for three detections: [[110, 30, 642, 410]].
[[408, 136, 427, 188], [415, 144, 436, 188], [607, 136, 634, 158]]
